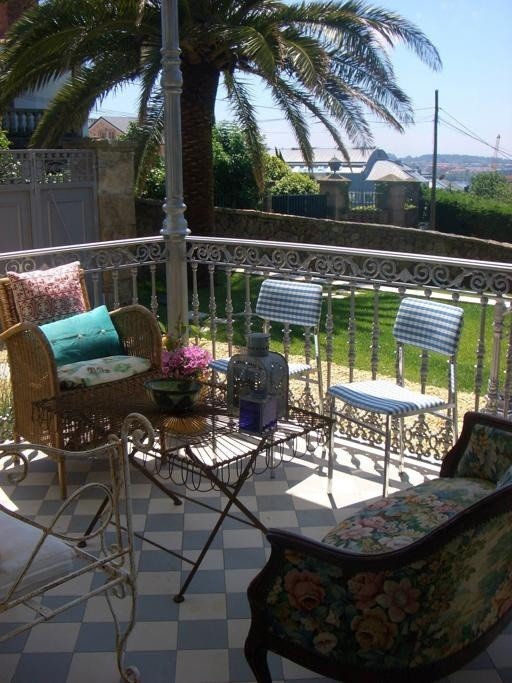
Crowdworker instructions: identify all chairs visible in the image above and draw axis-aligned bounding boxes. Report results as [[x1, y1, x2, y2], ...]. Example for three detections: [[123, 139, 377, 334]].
[[326, 297, 464, 498], [208, 279, 324, 479], [0, 268, 162, 501]]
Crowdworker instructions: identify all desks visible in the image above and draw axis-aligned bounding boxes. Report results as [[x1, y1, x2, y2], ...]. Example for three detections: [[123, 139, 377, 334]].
[[31, 370, 336, 603]]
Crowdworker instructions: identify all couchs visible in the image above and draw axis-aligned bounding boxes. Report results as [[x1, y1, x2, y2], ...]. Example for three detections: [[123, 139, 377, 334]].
[[245, 411, 512, 683]]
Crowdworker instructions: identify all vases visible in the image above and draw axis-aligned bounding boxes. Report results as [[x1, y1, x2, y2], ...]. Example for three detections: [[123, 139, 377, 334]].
[[164, 377, 209, 434]]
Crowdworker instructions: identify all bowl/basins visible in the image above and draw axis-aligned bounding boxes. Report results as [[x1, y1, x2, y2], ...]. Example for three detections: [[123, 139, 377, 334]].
[[143, 379, 203, 410]]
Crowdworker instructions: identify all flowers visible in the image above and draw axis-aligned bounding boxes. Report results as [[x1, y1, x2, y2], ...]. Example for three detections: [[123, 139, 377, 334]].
[[156, 310, 214, 379]]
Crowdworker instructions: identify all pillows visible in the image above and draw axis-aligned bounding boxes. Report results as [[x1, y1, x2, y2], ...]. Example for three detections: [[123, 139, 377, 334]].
[[6, 261, 86, 327], [39, 304, 123, 367]]
